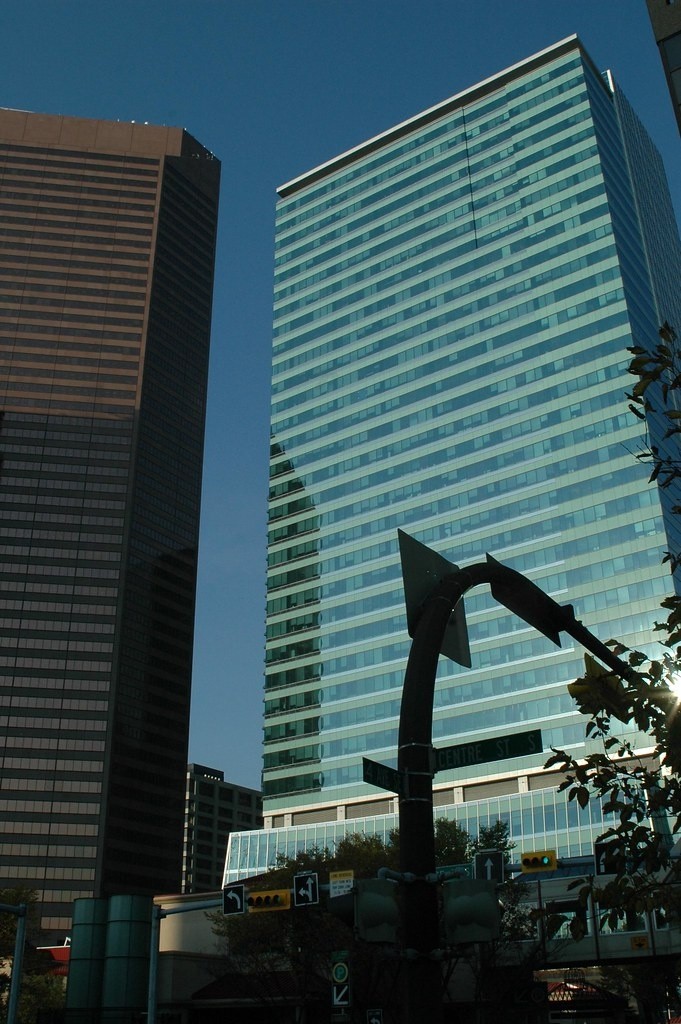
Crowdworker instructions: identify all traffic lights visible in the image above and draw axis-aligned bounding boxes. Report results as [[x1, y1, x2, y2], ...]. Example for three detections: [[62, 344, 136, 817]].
[[246, 888, 291, 912], [565, 651, 630, 727], [521, 849, 559, 873]]
